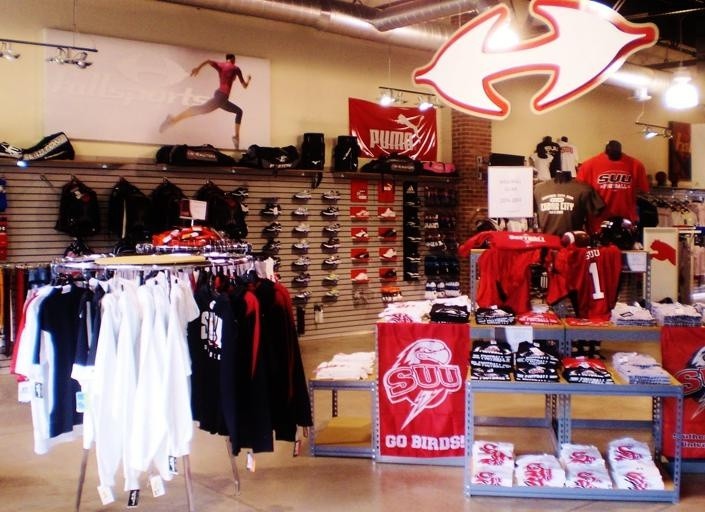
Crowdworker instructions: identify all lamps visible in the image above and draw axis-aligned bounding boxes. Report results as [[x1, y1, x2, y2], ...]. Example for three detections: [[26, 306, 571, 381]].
[[662, 127, 673, 140], [637, 123, 659, 146], [376, 92, 399, 109], [1, 37, 93, 69], [418, 101, 445, 115], [663, 13, 699, 110]]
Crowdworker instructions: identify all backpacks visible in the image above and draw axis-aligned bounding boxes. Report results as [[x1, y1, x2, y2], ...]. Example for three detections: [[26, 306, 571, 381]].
[[335, 136, 358, 172], [300, 133, 325, 170], [192, 180, 229, 230], [55, 176, 102, 238], [148, 176, 188, 234], [109, 178, 147, 237]]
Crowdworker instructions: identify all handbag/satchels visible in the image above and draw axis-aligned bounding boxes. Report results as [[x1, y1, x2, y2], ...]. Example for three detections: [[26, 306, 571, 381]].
[[422, 156, 458, 176], [239, 145, 299, 169], [361, 152, 422, 175], [155, 143, 238, 166]]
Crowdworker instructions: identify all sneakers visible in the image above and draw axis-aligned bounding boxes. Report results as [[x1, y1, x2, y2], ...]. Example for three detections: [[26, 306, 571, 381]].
[[294, 255, 311, 265], [232, 135, 239, 149], [230, 187, 248, 197], [356, 209, 369, 216], [325, 256, 342, 264], [159, 115, 174, 134], [294, 207, 308, 215], [294, 239, 310, 249], [323, 239, 341, 248], [322, 207, 339, 216], [385, 270, 396, 277], [262, 203, 282, 215], [324, 224, 341, 232], [324, 274, 339, 281], [240, 202, 249, 213], [381, 229, 396, 236], [295, 190, 311, 199], [263, 241, 281, 250], [383, 248, 397, 258], [381, 208, 396, 217], [264, 222, 282, 231], [295, 289, 313, 298], [355, 231, 368, 237], [326, 289, 340, 297], [323, 190, 341, 199], [354, 273, 368, 280], [295, 224, 310, 232], [295, 274, 311, 282], [355, 254, 369, 258]]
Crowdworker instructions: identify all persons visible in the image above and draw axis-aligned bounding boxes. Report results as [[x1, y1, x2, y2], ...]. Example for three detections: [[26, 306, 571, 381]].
[[531, 136, 578, 179], [159, 53, 251, 151], [533, 171, 605, 237], [577, 142, 650, 236]]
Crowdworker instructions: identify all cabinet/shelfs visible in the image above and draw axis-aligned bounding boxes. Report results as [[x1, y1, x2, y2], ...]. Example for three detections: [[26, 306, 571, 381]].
[[551, 297, 705, 473], [464, 243, 652, 311], [462, 355, 684, 503], [308, 299, 565, 501]]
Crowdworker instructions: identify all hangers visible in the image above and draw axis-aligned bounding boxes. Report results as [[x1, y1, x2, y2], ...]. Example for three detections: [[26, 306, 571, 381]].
[[47, 259, 263, 283], [649, 186, 705, 215], [141, 243, 248, 254]]
